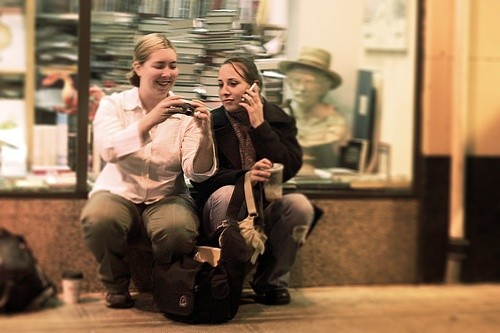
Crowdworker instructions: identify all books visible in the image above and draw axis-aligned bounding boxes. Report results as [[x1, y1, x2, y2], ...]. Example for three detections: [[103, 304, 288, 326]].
[[138, 1, 259, 31], [223, 32, 285, 105], [91, 2, 135, 93], [33, 112, 78, 188], [315, 70, 384, 188], [36, 1, 79, 65], [170, 33, 223, 112]]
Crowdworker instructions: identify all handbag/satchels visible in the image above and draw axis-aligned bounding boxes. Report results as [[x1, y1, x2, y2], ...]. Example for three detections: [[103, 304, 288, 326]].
[[153, 227, 244, 324], [217, 216, 268, 265], [222, 226, 256, 277]]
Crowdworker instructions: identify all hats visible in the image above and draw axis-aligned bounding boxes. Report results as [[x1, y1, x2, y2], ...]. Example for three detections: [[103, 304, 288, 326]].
[[278, 46, 342, 89]]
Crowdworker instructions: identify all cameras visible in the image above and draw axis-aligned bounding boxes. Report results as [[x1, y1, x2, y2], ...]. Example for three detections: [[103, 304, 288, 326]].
[[171, 101, 196, 115]]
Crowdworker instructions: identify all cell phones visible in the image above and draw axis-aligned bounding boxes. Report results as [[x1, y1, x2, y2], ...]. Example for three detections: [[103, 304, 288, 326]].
[[241, 83, 261, 102]]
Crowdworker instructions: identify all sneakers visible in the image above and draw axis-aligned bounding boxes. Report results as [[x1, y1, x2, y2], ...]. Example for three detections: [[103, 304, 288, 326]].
[[105, 290, 133, 308], [134, 292, 160, 312]]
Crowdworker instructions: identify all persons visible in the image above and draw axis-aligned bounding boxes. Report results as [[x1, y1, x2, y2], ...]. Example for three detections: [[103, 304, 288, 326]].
[[279, 45, 350, 169], [189, 56, 315, 305], [80, 32, 218, 308]]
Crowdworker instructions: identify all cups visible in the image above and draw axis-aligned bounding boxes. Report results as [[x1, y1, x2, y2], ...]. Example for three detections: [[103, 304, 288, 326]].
[[260, 163, 284, 201], [62, 272, 84, 304]]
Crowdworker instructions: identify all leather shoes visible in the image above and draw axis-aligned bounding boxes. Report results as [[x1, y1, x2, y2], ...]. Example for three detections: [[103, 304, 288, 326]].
[[250, 277, 289, 305]]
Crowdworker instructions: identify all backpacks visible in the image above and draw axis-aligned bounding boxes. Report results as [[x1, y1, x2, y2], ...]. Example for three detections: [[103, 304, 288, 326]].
[[0, 228, 56, 313]]
